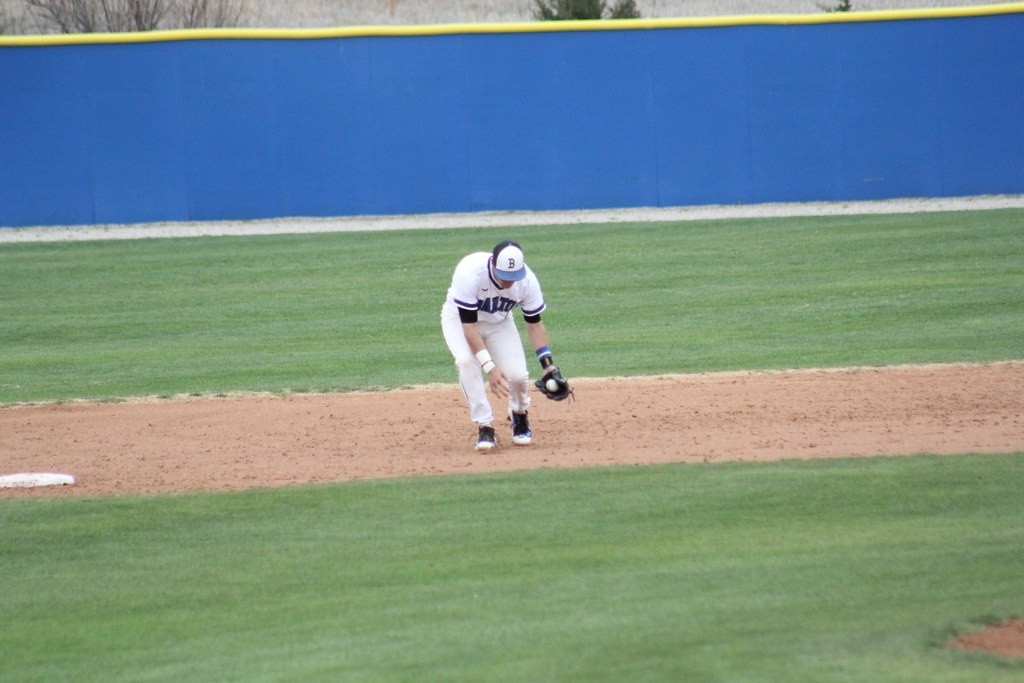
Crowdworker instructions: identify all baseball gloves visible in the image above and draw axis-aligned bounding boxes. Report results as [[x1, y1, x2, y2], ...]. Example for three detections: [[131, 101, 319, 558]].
[[535, 367, 570, 402]]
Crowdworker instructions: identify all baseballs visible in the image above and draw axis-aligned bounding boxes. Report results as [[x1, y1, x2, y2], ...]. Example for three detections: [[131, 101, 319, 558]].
[[545, 379, 559, 392]]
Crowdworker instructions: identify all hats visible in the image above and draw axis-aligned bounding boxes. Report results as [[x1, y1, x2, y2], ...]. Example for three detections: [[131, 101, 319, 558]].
[[492, 241, 526, 281]]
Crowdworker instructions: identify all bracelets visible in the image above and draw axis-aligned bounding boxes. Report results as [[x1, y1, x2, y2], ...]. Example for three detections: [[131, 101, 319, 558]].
[[535, 346, 553, 370], [475, 349, 496, 375]]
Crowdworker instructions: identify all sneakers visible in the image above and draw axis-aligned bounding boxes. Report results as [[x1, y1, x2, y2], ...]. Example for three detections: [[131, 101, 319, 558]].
[[508, 408, 531, 446], [474, 425, 496, 450]]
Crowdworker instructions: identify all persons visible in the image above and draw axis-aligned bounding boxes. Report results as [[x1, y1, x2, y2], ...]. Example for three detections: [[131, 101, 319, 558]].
[[441, 240, 569, 453]]
